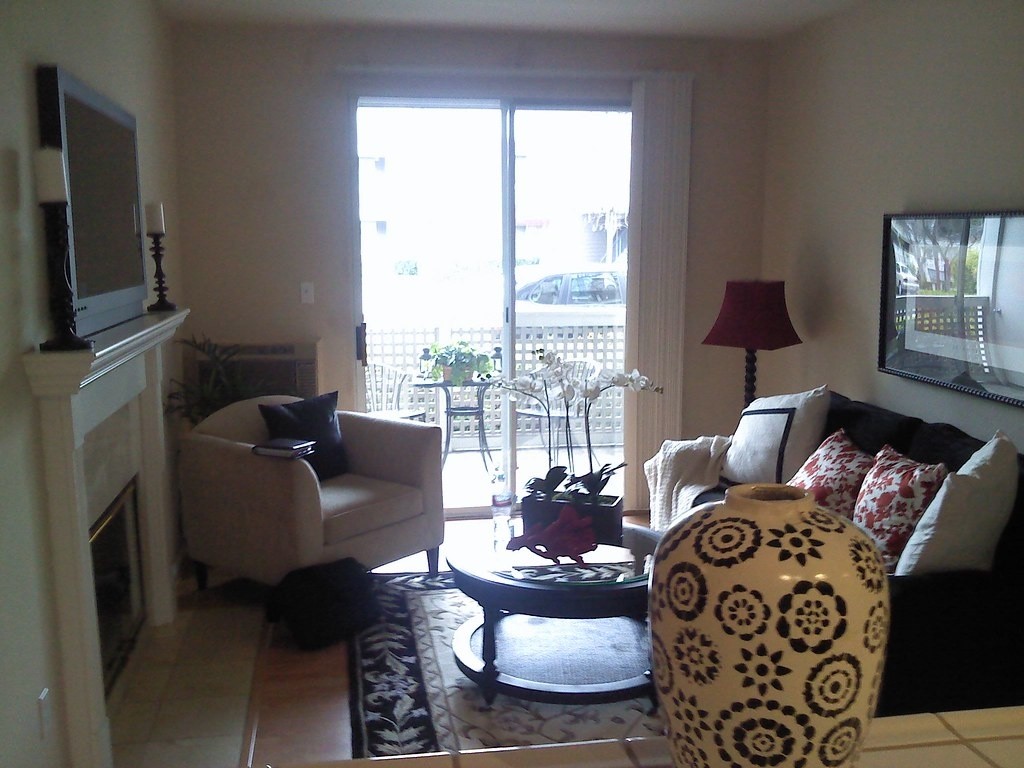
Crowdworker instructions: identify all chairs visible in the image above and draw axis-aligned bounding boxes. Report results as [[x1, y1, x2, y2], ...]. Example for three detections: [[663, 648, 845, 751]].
[[364, 360, 426, 422], [515, 359, 603, 468], [178, 395, 444, 619]]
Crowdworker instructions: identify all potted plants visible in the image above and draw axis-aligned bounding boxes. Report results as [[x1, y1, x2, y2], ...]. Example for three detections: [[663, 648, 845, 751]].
[[521, 462, 627, 546], [420, 342, 494, 388]]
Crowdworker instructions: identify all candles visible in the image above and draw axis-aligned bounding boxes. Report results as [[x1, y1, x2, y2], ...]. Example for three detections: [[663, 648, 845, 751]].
[[34, 146, 69, 203], [146, 202, 164, 234]]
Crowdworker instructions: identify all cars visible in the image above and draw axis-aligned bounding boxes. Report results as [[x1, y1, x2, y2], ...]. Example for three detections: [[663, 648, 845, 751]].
[[515, 272, 626, 306], [895, 262, 919, 296]]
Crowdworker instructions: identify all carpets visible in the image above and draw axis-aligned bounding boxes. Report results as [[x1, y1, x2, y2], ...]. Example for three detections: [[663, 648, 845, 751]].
[[346, 560, 648, 759]]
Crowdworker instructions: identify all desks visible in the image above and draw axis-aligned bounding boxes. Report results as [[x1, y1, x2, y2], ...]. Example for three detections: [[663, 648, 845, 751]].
[[412, 376, 503, 483]]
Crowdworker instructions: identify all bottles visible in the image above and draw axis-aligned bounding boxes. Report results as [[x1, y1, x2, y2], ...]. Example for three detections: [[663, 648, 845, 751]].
[[491, 346, 503, 373], [420, 348, 433, 373], [492, 474, 513, 543]]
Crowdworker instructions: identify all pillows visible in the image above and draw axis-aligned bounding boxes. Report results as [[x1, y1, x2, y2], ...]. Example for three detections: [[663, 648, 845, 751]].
[[257, 392, 353, 481], [718, 384, 831, 484], [895, 429, 1018, 577], [851, 442, 946, 574], [788, 427, 874, 521]]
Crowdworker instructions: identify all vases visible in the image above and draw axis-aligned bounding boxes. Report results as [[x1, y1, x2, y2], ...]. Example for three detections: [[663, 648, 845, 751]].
[[877, 211, 1024, 409], [649, 484, 889, 768]]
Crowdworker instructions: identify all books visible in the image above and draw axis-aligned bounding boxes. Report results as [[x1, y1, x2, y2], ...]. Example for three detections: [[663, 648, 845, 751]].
[[251, 438, 316, 459]]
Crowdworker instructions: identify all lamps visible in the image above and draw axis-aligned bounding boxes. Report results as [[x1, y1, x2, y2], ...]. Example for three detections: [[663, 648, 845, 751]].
[[700, 279, 802, 406]]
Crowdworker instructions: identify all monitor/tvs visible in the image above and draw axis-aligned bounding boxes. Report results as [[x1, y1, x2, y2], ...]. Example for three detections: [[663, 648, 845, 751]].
[[35, 64, 148, 337]]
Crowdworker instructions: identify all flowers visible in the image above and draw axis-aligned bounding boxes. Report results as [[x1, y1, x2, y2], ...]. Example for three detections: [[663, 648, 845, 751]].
[[478, 349, 664, 472]]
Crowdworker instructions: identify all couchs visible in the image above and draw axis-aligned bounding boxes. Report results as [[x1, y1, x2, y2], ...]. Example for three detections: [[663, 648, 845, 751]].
[[643, 389, 1024, 718]]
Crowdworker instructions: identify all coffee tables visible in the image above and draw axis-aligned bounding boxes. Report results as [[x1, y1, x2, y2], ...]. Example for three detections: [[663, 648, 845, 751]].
[[445, 521, 662, 717]]
